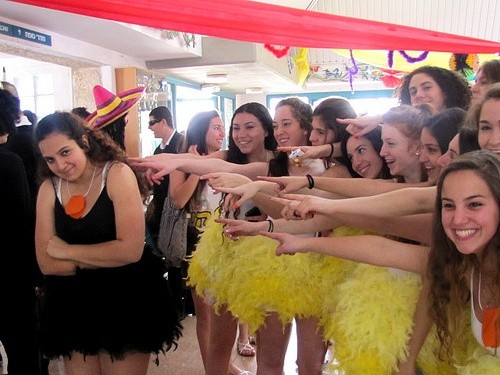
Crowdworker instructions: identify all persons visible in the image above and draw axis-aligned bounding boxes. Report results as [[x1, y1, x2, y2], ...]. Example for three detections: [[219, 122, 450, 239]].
[[259, 149, 499, 374], [0, 89, 22, 124], [148, 106, 185, 245], [128, 84, 500, 374], [0, 112, 51, 375], [158, 108, 225, 266], [86, 85, 164, 196], [336, 65, 473, 137], [33, 111, 187, 375], [472, 60, 500, 101]]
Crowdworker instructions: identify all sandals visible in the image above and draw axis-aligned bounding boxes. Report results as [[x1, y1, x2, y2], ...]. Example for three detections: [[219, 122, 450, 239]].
[[229, 369, 254, 375], [236, 338, 256, 357], [248, 335, 257, 344]]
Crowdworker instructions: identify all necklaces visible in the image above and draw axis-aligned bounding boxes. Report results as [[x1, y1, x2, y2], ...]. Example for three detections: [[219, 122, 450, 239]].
[[482, 288, 494, 308], [67, 165, 96, 199]]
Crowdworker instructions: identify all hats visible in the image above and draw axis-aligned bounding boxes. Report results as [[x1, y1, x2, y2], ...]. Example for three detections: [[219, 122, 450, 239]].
[[83, 85, 146, 132]]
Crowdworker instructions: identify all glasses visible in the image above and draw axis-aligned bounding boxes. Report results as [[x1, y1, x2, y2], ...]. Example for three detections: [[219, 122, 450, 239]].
[[149, 119, 161, 126]]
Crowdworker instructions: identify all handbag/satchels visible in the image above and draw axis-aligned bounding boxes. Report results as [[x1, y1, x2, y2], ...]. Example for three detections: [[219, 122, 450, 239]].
[[157, 196, 188, 268]]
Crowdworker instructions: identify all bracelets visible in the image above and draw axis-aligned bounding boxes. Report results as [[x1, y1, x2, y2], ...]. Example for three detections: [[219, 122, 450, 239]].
[[268, 219, 274, 232], [306, 174, 314, 189], [326, 143, 334, 158]]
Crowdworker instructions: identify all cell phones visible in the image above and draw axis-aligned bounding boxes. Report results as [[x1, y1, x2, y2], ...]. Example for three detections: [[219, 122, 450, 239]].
[[289, 149, 311, 168], [245, 207, 261, 222]]
